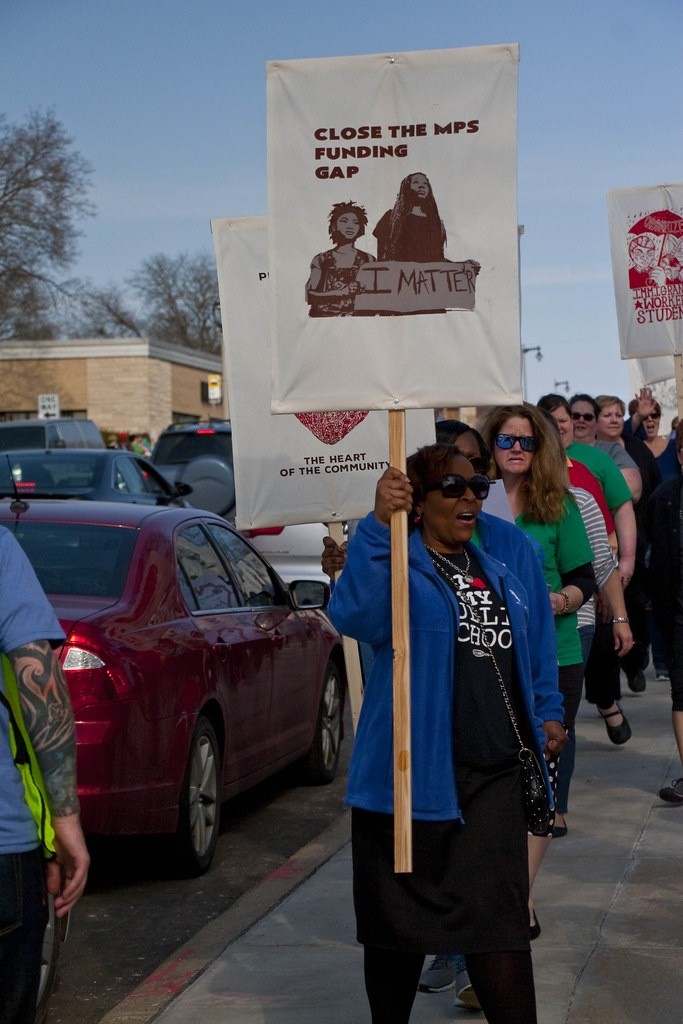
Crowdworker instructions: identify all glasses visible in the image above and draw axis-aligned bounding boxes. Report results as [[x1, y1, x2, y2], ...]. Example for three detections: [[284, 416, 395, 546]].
[[494, 433, 537, 453], [642, 413, 660, 421], [468, 457, 490, 475], [425, 474, 490, 500], [570, 412, 596, 422]]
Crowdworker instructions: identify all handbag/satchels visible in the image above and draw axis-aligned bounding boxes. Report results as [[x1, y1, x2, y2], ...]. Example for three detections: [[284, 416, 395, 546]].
[[518, 748, 554, 838]]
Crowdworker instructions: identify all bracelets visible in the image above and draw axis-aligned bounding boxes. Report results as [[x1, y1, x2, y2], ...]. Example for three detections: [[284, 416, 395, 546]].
[[613, 617, 629, 623], [556, 591, 571, 615]]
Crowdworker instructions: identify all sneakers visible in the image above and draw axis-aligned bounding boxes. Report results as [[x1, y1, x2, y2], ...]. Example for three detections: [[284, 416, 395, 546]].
[[452, 968, 482, 1010], [659, 778, 683, 802], [417, 954, 466, 990]]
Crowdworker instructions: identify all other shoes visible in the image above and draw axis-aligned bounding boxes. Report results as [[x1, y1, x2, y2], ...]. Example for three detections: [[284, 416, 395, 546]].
[[621, 665, 646, 693], [554, 817, 567, 837], [529, 908, 541, 941], [654, 670, 670, 681], [597, 702, 631, 745]]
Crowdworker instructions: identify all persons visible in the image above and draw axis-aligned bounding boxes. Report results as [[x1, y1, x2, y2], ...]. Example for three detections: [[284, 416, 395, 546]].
[[537, 409, 632, 838], [537, 387, 678, 745], [649, 421, 683, 804], [471, 400, 599, 941], [0, 525, 88, 1024], [106, 432, 152, 476], [322, 418, 568, 1014], [332, 445, 571, 1024]]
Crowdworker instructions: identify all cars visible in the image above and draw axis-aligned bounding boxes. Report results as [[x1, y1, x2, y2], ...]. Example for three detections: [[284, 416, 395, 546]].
[[0, 449, 206, 574], [0, 496, 353, 883]]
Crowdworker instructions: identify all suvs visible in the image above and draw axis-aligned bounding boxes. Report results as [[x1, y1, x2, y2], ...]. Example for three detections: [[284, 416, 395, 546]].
[[141, 419, 236, 523]]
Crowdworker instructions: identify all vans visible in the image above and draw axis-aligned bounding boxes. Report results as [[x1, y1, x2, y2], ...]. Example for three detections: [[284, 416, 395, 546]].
[[0, 415, 111, 485]]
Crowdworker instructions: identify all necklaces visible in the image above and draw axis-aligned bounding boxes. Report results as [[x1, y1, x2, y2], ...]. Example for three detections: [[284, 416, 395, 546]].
[[424, 544, 474, 584]]
